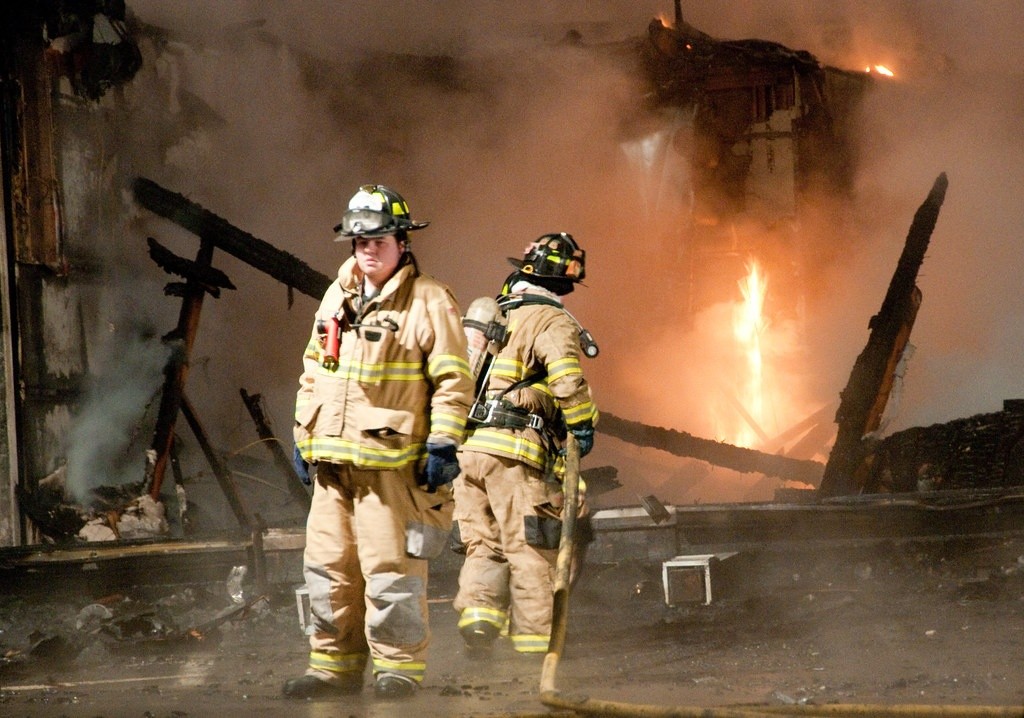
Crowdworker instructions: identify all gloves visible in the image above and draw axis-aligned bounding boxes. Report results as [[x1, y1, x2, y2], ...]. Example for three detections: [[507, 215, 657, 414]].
[[558, 420, 594, 459], [418, 444, 461, 493], [292, 444, 311, 487]]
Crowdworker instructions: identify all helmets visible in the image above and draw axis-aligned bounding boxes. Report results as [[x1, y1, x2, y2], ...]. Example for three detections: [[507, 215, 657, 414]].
[[333, 185, 430, 241], [507, 232, 586, 283]]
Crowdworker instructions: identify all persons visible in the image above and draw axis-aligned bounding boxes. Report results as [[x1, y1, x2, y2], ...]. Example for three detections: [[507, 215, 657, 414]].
[[452, 232, 600, 654], [282, 184, 474, 698]]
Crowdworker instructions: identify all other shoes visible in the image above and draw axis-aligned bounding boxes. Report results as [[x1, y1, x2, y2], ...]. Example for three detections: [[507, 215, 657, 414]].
[[375, 677, 412, 698], [283, 675, 364, 699], [466, 631, 493, 658]]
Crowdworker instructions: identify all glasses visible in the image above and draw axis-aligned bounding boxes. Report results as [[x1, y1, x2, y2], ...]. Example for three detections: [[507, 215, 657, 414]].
[[341, 210, 392, 235]]
